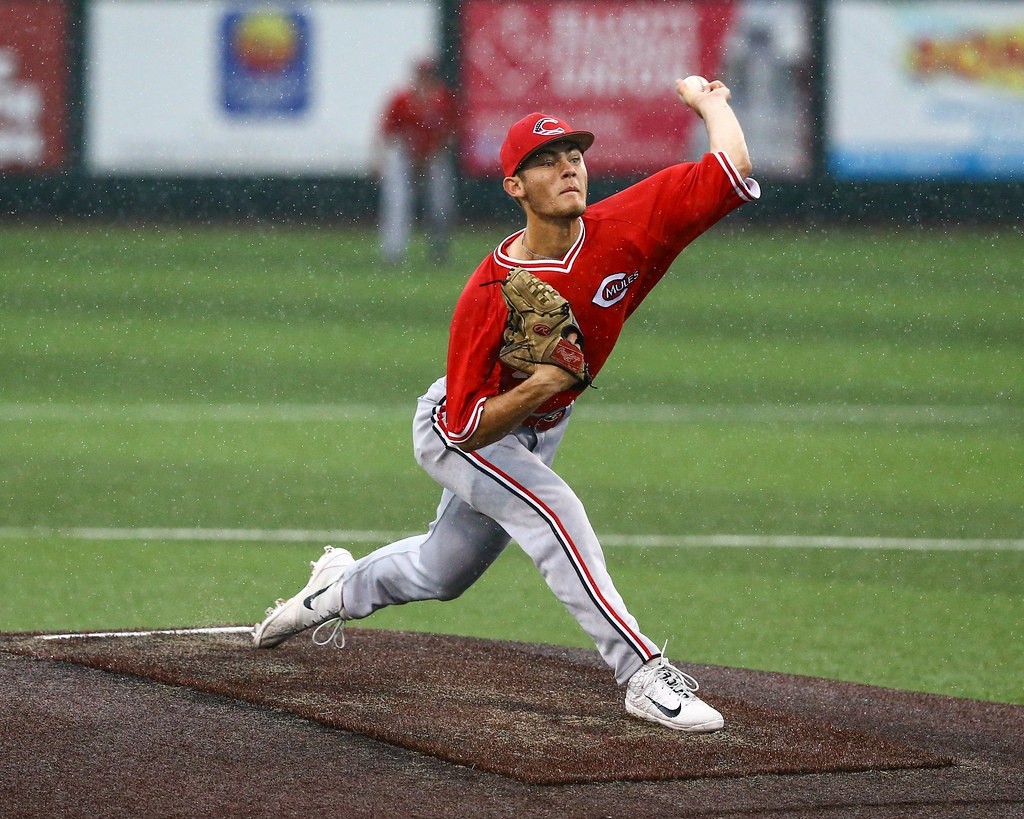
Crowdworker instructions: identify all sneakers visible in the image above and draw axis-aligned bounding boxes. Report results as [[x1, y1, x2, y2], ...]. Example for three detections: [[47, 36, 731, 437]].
[[625, 638, 724, 732], [251, 545, 356, 649]]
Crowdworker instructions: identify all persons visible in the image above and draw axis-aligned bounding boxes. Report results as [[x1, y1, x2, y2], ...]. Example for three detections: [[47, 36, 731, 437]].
[[374, 58, 460, 269], [251, 77, 760, 734]]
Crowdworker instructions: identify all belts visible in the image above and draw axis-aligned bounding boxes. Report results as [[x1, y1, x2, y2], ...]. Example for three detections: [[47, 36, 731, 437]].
[[521, 407, 566, 434]]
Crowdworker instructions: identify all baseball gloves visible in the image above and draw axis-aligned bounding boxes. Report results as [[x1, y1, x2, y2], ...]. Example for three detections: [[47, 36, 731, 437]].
[[476, 265, 599, 391]]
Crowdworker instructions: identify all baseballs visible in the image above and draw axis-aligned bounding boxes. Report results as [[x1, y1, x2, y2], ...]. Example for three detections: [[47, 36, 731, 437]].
[[678, 75, 711, 104]]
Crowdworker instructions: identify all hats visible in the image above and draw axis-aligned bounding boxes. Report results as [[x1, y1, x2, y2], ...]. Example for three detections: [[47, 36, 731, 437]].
[[499, 112, 595, 177]]
